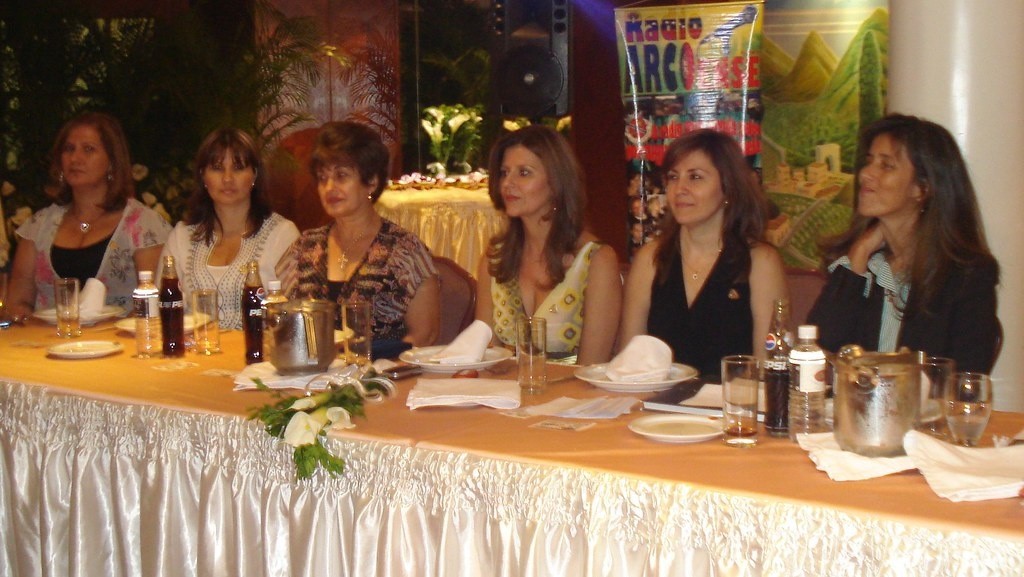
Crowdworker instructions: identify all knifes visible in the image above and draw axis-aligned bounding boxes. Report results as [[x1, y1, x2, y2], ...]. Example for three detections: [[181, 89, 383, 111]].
[[95, 326, 118, 331], [548, 375, 577, 382]]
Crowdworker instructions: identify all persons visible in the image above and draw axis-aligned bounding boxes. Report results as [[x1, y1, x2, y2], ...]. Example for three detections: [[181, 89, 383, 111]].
[[4, 112, 165, 326], [806, 114, 1004, 403], [470, 126, 624, 371], [266, 122, 443, 347], [609, 128, 791, 380], [150, 124, 304, 338]]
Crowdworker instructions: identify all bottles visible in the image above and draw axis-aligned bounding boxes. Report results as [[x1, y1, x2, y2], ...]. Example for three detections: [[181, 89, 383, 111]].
[[240, 260, 266, 365], [132, 270, 162, 359], [763, 298, 796, 439], [261, 280, 288, 361], [157, 255, 185, 358], [786, 324, 827, 444]]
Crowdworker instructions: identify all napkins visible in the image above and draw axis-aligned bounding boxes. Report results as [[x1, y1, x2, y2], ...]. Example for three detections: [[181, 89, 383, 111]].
[[602, 333, 674, 383], [402, 375, 524, 413], [430, 317, 495, 366], [231, 356, 361, 394], [790, 422, 1024, 505], [73, 275, 109, 323]]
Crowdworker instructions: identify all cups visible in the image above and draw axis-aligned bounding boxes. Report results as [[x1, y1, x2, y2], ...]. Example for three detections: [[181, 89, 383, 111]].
[[191, 289, 219, 356], [914, 355, 955, 441], [516, 317, 547, 394], [720, 355, 761, 449], [0, 272, 14, 329], [53, 277, 82, 338], [944, 371, 994, 447], [341, 301, 371, 368]]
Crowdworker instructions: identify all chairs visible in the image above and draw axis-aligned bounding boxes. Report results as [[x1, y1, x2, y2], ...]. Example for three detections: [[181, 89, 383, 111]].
[[417, 246, 483, 352]]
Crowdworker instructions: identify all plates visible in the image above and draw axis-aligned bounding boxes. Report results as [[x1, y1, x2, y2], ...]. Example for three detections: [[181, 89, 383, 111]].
[[628, 413, 723, 443], [400, 344, 513, 373], [334, 329, 354, 345], [824, 397, 944, 424], [32, 305, 124, 325], [573, 362, 698, 392], [113, 313, 211, 335], [46, 341, 124, 359]]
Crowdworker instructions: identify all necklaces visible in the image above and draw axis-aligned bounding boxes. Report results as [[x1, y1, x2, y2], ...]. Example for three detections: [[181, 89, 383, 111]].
[[684, 242, 723, 280], [73, 202, 108, 233], [336, 211, 377, 269]]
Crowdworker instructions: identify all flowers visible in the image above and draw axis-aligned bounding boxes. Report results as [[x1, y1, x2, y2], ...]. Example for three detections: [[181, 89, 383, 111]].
[[416, 101, 469, 181], [240, 369, 368, 486]]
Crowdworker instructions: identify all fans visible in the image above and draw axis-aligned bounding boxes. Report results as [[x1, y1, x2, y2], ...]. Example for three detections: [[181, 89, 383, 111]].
[[488, 44, 566, 128]]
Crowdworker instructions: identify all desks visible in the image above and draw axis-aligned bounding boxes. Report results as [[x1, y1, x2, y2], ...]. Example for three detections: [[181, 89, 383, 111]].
[[0, 310, 1024, 577], [367, 177, 514, 347]]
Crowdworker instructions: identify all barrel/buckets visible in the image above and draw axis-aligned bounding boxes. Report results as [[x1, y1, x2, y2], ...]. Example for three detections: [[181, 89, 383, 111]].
[[827, 348, 925, 459], [264, 298, 338, 376]]
